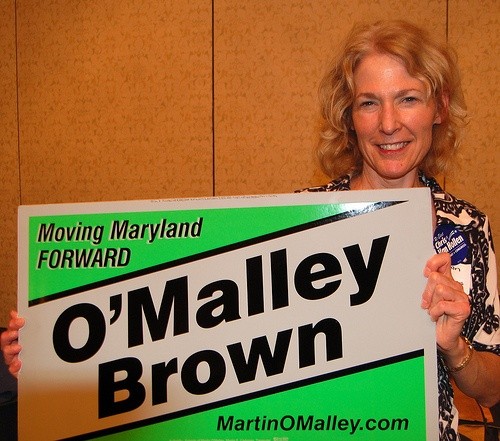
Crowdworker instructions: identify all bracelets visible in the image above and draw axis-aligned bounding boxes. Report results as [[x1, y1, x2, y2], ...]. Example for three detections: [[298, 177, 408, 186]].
[[442, 335, 474, 373]]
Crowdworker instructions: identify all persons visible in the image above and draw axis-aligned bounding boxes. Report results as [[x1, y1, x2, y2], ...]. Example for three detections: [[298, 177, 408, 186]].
[[2, 19, 500, 441]]
